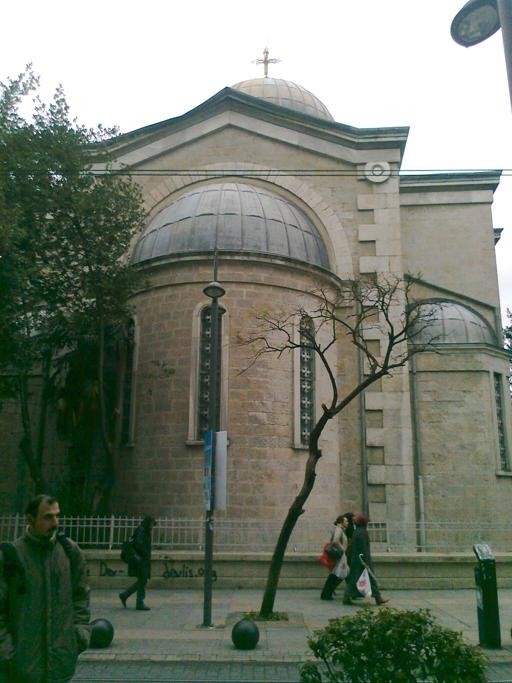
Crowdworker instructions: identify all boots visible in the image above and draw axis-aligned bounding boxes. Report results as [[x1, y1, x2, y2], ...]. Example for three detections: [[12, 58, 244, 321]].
[[376, 596, 391, 605], [343, 594, 357, 605], [119, 589, 131, 609], [136, 600, 151, 610]]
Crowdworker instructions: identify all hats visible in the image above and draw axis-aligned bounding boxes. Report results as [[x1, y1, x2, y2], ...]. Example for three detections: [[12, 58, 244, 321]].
[[353, 512, 368, 524]]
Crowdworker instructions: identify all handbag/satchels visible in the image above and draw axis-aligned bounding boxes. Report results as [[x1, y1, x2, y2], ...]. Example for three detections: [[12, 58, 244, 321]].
[[120, 541, 142, 566], [320, 543, 344, 572]]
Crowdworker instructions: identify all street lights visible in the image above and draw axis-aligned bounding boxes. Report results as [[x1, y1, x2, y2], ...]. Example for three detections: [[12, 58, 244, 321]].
[[204, 283, 225, 627]]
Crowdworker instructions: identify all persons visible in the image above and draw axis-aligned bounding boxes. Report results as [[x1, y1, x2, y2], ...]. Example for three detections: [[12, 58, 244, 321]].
[[118, 514, 157, 610], [321, 515, 352, 599], [341, 512, 366, 598], [0, 493, 92, 682], [344, 515, 391, 605]]
